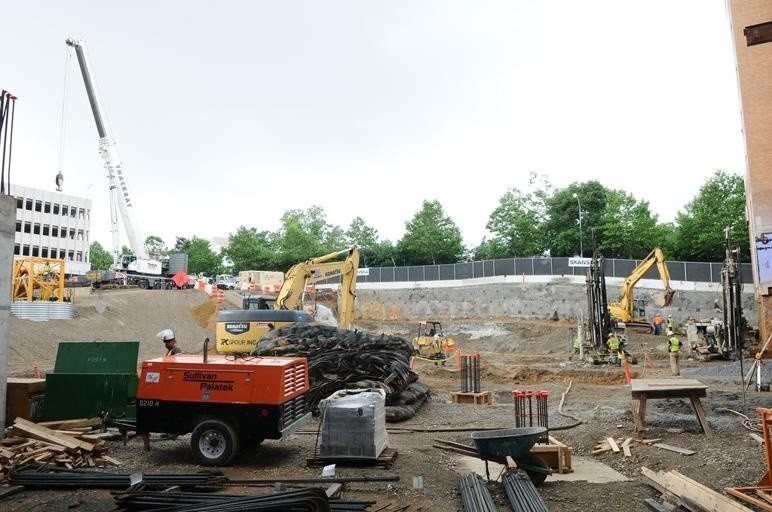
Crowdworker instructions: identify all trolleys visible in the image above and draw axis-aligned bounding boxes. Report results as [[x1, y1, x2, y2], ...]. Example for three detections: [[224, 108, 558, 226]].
[[432, 426, 551, 487]]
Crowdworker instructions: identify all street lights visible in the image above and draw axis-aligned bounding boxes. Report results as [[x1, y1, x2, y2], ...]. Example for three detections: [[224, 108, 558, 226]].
[[570, 193, 583, 259]]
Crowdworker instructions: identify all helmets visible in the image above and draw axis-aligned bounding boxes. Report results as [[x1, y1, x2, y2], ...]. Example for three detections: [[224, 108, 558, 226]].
[[157, 329, 175, 341], [608, 313, 674, 336]]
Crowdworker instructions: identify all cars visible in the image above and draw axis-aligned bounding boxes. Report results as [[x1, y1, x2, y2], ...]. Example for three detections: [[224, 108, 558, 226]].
[[187, 272, 239, 289]]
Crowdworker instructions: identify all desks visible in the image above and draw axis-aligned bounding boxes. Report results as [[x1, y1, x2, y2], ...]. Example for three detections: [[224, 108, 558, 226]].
[[628, 378, 714, 440]]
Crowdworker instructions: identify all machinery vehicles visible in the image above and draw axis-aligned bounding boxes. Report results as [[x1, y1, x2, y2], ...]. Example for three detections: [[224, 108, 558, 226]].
[[56, 36, 176, 290], [412, 321, 445, 358], [608, 248, 675, 335], [217, 243, 361, 355]]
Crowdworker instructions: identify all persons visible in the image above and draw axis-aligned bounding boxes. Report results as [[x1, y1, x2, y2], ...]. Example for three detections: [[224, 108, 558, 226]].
[[29, 359, 43, 379], [157, 325, 184, 359], [42, 260, 52, 282], [666, 331, 683, 377], [569, 334, 583, 362], [427, 339, 442, 356], [605, 332, 620, 364], [683, 315, 701, 363], [652, 312, 663, 338], [666, 312, 674, 331]]
